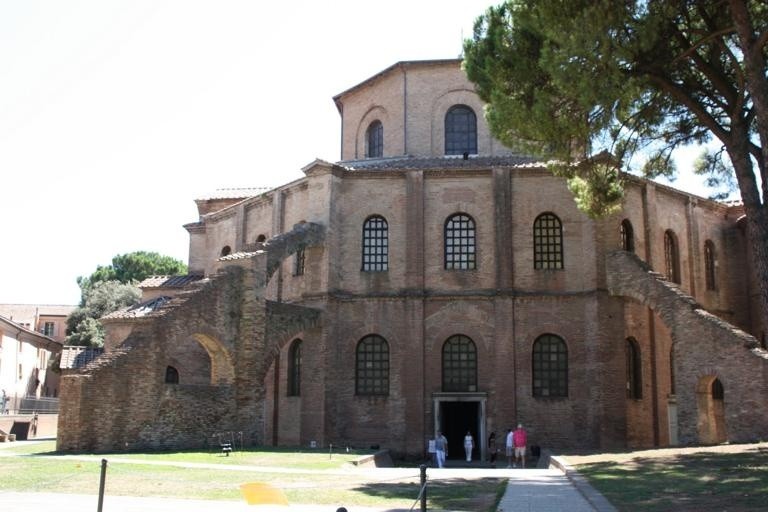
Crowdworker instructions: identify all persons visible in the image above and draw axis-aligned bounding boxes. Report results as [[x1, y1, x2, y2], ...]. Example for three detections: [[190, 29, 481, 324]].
[[506, 431, 514, 469], [488, 432, 496, 466], [464, 432, 475, 463], [428, 436, 436, 467], [512, 423, 527, 469], [435, 430, 448, 468]]
